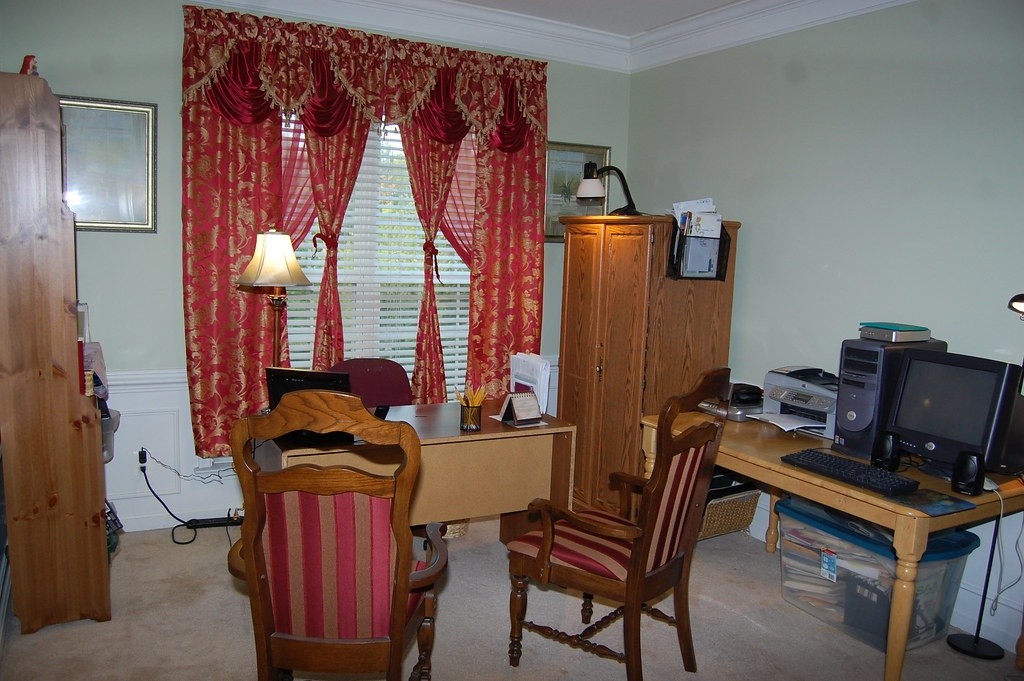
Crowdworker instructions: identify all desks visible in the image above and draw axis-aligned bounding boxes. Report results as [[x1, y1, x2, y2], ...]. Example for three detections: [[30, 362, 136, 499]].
[[641, 410, 1024, 681], [251, 398, 577, 526]]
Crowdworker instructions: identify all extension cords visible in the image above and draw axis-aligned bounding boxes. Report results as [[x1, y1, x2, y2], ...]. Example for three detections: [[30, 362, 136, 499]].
[[187, 516, 244, 528]]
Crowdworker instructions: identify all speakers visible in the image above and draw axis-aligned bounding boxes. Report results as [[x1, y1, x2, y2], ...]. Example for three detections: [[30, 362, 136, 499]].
[[950, 448, 986, 496], [871, 430, 900, 472]]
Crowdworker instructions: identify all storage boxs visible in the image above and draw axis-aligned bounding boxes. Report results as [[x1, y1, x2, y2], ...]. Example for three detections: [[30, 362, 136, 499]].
[[774, 493, 981, 652]]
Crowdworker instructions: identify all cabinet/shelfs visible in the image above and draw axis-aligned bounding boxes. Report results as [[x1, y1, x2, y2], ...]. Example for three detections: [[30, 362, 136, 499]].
[[554, 216, 742, 539], [0, 71, 112, 633]]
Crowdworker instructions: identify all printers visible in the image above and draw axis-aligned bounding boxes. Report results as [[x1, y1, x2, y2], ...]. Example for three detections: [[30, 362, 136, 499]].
[[746, 365, 839, 440]]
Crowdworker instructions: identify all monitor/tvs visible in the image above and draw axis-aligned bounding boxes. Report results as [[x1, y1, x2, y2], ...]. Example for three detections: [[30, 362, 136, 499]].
[[266, 365, 355, 444], [885, 347, 1024, 476]]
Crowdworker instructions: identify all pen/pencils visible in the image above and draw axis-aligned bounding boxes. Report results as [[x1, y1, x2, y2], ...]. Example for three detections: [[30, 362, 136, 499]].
[[454, 384, 488, 431], [684, 211, 693, 235]]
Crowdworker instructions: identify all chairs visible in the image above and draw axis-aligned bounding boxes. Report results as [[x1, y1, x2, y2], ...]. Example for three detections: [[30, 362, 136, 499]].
[[230, 389, 448, 681], [329, 358, 416, 406], [507, 366, 732, 681]]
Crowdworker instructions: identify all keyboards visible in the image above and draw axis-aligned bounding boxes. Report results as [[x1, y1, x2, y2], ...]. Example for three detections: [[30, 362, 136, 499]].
[[781, 448, 921, 498]]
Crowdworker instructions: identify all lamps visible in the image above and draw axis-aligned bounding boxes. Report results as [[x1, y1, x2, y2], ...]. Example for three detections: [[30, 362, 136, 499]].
[[946, 293, 1024, 660], [236, 221, 312, 367], [576, 160, 642, 216]]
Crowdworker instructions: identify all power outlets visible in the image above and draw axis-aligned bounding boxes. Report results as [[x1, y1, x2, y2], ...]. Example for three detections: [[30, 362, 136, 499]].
[[134, 451, 150, 476]]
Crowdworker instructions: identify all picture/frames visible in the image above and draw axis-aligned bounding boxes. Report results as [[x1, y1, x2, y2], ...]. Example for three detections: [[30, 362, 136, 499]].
[[57, 94, 158, 234], [544, 140, 611, 243]]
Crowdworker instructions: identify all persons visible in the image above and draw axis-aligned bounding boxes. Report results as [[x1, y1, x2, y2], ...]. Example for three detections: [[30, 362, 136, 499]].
[[998, 621, 1024, 681]]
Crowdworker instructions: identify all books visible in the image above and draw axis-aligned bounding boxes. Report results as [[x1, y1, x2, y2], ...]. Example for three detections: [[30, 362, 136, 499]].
[[780, 517, 948, 623], [672, 199, 722, 278]]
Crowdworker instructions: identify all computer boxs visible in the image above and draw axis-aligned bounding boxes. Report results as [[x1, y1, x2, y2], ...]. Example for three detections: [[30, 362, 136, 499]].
[[831, 337, 948, 460]]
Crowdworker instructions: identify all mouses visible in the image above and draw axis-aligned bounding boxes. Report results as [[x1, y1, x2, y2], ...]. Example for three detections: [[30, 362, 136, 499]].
[[983, 476, 999, 490]]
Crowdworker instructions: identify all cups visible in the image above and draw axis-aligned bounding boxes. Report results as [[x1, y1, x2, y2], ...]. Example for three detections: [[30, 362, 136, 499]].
[[460, 406, 481, 431]]
